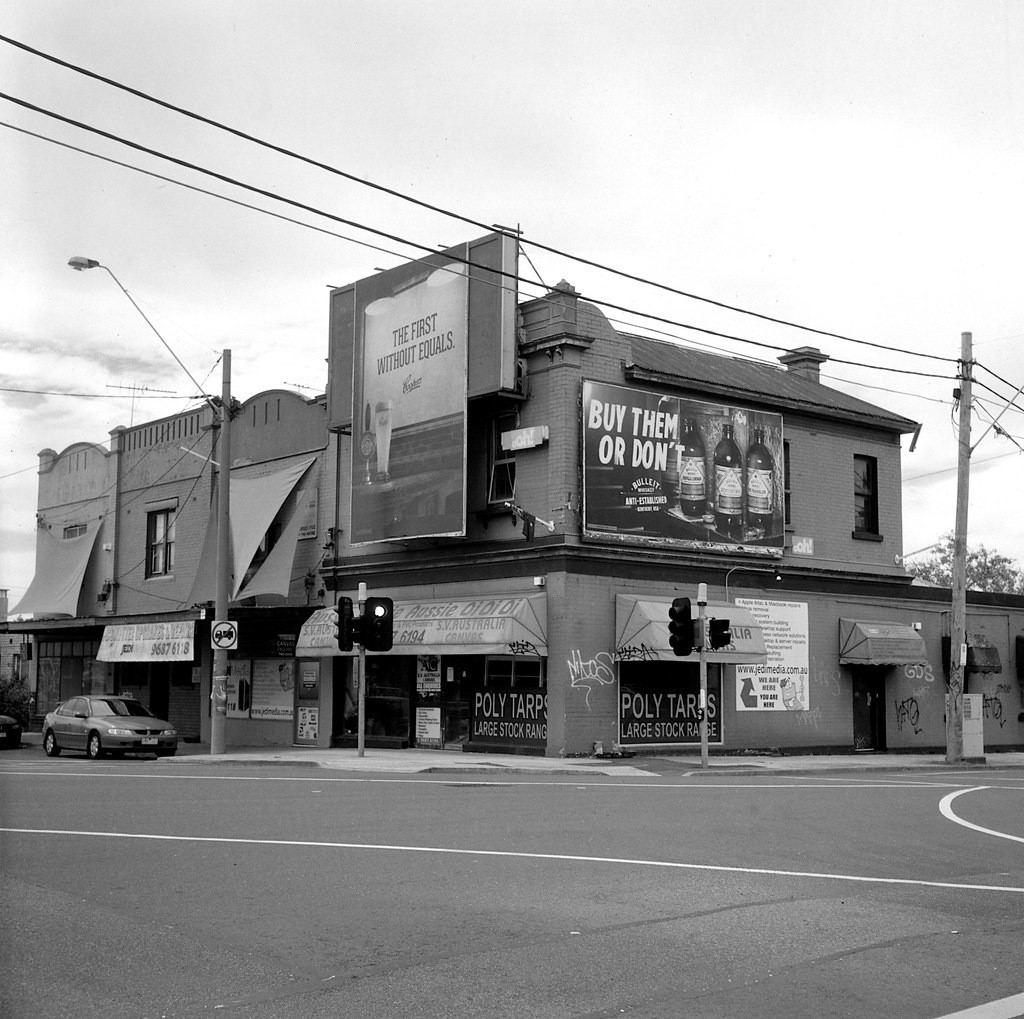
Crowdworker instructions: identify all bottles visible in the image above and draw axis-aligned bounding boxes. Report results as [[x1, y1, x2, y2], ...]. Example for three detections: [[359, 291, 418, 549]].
[[746, 429, 775, 530], [679, 418, 708, 518], [713, 423, 744, 532]]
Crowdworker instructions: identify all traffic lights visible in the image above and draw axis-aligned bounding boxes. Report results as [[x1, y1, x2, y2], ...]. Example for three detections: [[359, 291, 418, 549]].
[[709, 618, 730, 650], [332, 596, 354, 652], [669, 597, 692, 657], [364, 597, 394, 652]]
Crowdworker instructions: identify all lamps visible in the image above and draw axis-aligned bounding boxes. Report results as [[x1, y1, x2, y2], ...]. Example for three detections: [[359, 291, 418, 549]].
[[773, 569, 782, 581]]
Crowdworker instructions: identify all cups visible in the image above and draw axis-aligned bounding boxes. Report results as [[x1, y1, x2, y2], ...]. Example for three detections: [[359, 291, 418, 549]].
[[374, 402, 392, 483]]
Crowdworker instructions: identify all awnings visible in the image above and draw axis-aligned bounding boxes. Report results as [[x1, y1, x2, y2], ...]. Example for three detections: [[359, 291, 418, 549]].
[[955, 633, 1003, 674], [614, 592, 768, 663], [837, 617, 927, 668], [295, 591, 550, 659]]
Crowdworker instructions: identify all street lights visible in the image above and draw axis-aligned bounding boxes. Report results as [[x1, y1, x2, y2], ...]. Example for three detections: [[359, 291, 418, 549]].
[[68, 256, 233, 757]]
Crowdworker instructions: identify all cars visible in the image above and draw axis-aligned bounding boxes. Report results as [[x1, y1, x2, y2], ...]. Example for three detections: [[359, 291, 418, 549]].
[[0, 715, 22, 750], [41, 695, 178, 758]]
